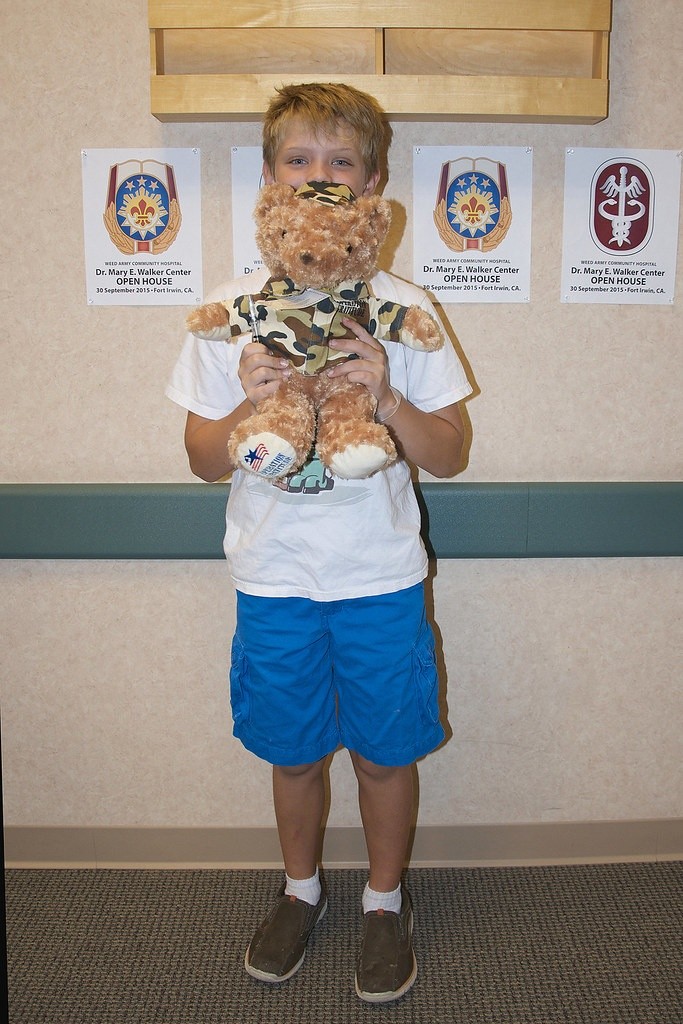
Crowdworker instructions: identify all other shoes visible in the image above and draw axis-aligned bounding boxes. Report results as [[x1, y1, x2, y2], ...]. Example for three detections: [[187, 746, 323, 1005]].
[[244, 863, 328, 983], [354, 887, 419, 1003]]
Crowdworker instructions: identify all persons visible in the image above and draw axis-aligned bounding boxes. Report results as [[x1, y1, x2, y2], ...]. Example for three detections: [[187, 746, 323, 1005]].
[[165, 83, 474, 1003]]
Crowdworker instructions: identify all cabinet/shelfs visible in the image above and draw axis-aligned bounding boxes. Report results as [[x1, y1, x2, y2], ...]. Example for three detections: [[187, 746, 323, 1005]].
[[146, 0, 614, 126]]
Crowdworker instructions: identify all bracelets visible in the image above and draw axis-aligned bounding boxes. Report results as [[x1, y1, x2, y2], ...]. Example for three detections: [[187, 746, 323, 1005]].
[[378, 386, 403, 422]]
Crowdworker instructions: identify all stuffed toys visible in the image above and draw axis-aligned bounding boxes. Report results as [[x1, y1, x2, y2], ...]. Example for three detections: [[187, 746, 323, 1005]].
[[185, 182, 443, 482]]
